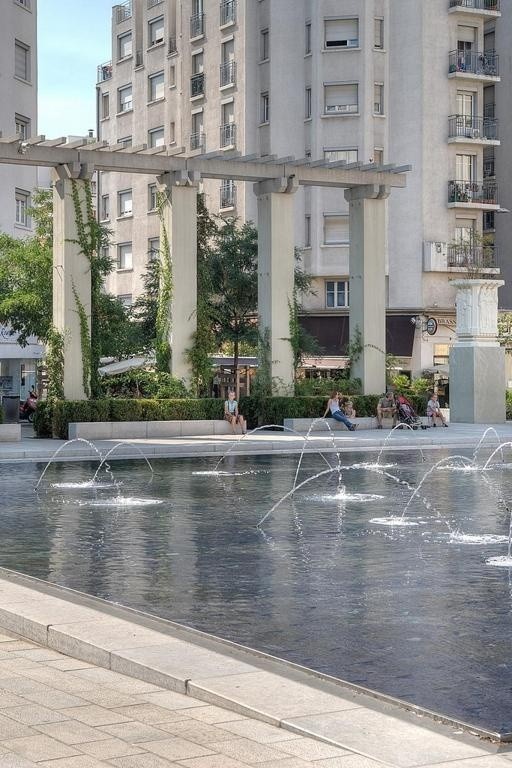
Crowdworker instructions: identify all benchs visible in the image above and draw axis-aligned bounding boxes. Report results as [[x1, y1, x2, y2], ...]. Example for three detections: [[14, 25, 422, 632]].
[[68, 419, 249, 442], [283, 415, 444, 432]]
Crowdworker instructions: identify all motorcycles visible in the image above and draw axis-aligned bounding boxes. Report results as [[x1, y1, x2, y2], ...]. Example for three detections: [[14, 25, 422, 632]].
[[20, 385, 37, 422]]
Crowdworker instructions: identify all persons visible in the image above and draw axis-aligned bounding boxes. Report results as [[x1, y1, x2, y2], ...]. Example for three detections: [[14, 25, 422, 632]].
[[224, 391, 247, 435], [376, 391, 398, 429], [321, 391, 359, 431], [427, 394, 449, 427]]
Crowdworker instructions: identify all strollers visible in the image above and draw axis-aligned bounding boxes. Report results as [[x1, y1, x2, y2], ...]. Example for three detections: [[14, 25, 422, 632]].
[[395, 392, 427, 429]]
